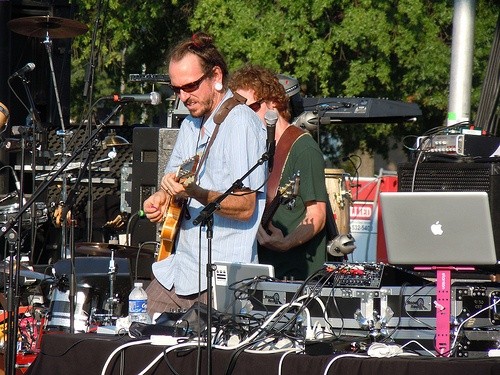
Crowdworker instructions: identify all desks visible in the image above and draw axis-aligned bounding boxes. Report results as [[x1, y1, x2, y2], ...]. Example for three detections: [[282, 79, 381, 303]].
[[25, 332, 500, 375]]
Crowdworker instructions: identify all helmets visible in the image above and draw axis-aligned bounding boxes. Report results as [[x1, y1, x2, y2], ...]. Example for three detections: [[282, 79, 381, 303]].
[[273, 74, 300, 97]]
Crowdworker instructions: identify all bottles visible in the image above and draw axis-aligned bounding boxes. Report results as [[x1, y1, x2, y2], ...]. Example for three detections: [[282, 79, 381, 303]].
[[128, 283, 148, 338]]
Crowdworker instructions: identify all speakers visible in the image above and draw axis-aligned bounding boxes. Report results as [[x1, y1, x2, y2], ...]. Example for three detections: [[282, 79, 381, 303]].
[[131, 127, 181, 250]]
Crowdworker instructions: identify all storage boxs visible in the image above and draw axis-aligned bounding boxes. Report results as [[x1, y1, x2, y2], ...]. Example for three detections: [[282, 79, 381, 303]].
[[241, 280, 500, 360]]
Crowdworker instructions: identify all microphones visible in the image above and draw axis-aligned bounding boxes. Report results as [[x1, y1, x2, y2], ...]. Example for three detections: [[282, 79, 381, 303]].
[[264, 110, 279, 174], [9, 62, 36, 79], [104, 91, 161, 106]]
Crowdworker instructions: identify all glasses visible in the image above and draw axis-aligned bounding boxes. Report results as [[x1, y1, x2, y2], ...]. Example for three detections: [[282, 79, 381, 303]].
[[247, 95, 270, 112], [168, 67, 212, 94]]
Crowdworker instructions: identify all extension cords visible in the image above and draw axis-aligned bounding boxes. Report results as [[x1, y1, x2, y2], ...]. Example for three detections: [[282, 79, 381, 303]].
[[150, 334, 206, 346]]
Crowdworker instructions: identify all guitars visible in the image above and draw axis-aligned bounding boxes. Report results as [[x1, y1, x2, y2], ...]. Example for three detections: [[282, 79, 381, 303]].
[[156, 155, 200, 262], [260, 169, 300, 230]]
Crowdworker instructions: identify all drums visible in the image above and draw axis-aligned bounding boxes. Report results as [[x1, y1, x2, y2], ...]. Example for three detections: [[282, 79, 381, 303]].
[[44, 283, 93, 334]]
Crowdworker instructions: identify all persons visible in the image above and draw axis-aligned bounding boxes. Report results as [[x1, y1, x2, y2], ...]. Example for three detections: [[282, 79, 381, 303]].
[[229, 65, 328, 281], [143, 40, 267, 319]]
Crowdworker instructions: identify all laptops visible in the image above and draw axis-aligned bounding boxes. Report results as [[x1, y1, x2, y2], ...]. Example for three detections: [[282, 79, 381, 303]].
[[213, 261, 275, 315], [379, 191, 500, 265]]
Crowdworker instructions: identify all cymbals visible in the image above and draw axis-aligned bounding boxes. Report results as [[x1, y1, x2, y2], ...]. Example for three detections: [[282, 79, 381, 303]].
[[76, 243, 153, 266]]
